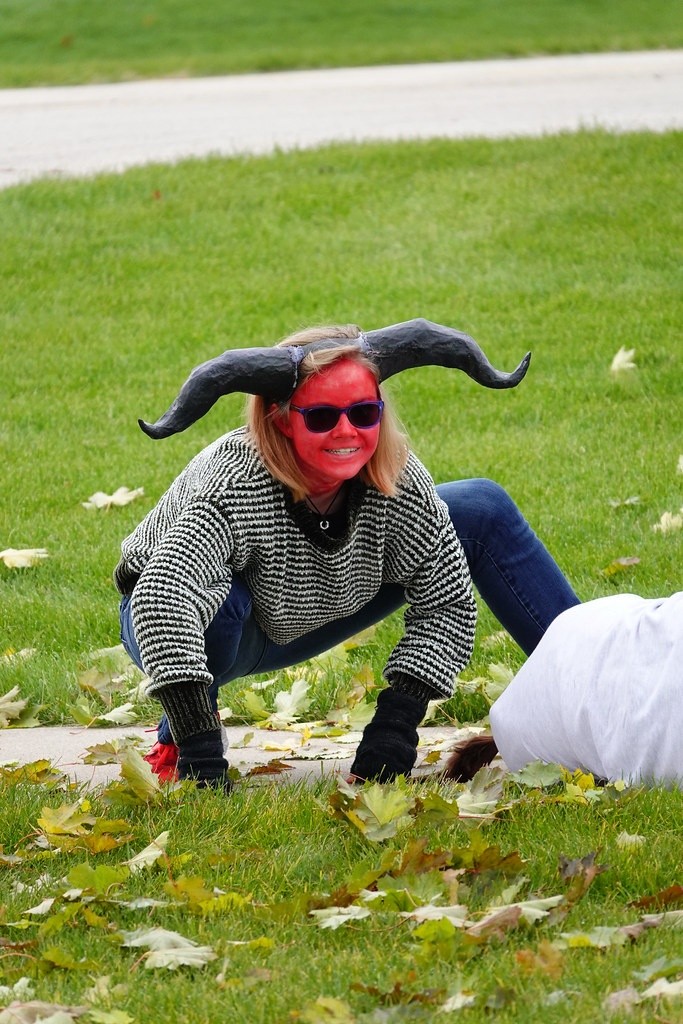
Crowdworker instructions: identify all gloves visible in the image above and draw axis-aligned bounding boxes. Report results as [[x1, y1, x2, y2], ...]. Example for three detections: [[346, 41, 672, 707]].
[[351, 686, 426, 784], [177, 728, 231, 798]]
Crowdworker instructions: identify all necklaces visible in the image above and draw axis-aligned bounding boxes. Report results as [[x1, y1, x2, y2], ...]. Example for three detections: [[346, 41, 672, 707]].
[[304, 488, 341, 530]]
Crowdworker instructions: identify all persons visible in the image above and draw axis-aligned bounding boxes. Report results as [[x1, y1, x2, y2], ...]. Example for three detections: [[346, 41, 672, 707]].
[[447, 594, 683, 785], [115, 317, 587, 792]]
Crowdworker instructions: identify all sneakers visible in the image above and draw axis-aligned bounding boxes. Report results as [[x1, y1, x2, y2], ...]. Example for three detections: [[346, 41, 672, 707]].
[[143, 712, 230, 790]]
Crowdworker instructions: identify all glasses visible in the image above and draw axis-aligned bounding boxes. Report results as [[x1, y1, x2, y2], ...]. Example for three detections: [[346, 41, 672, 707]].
[[277, 399, 384, 434]]
